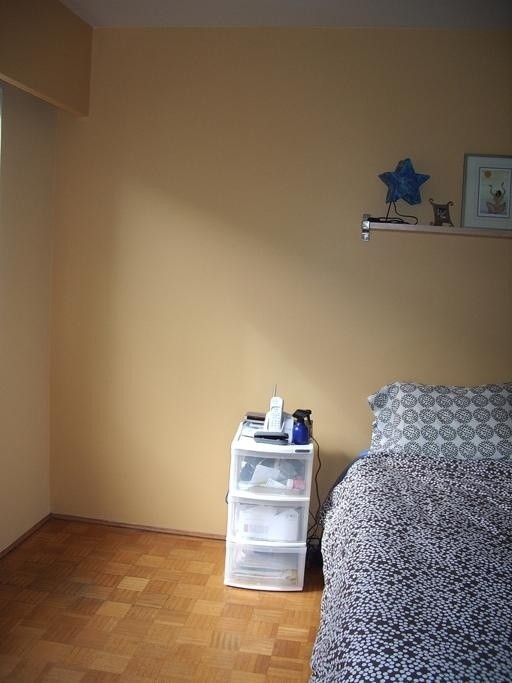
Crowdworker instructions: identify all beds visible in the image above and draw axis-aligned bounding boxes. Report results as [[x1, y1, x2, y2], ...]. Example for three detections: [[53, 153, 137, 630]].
[[306, 437, 511, 680]]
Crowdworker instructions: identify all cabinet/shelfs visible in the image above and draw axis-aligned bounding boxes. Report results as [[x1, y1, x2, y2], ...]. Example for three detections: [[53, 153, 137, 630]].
[[221, 420, 317, 591]]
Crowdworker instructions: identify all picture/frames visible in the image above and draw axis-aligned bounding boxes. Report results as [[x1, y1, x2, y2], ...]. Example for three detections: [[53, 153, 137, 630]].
[[458, 151, 511, 231]]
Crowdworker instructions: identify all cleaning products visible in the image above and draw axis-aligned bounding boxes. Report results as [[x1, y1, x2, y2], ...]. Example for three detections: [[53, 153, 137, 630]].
[[292, 409, 312, 445]]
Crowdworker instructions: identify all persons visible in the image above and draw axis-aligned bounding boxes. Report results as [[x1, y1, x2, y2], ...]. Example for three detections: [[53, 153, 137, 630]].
[[487, 182, 506, 214]]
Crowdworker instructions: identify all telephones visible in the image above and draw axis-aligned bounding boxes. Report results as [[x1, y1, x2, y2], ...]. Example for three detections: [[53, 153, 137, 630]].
[[263, 384, 285, 433]]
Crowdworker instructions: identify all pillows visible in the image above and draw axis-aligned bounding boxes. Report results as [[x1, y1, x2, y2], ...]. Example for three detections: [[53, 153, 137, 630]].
[[363, 383, 512, 464]]
